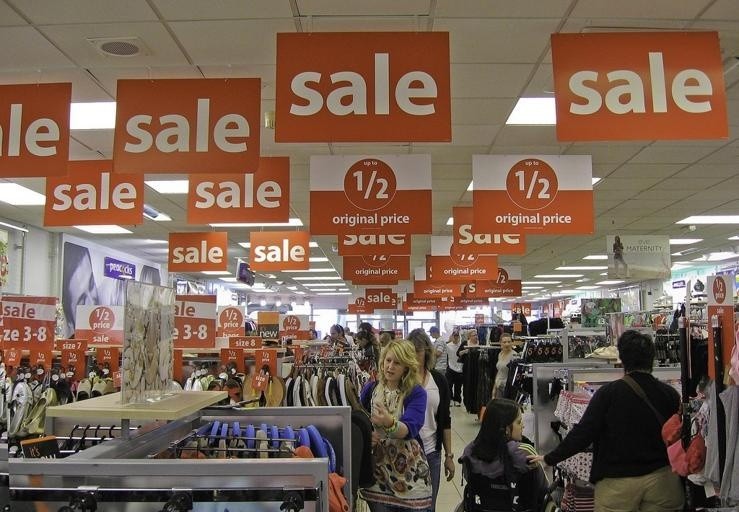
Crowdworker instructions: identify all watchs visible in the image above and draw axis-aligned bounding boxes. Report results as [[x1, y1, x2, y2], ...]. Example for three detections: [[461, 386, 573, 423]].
[[445, 453, 454, 459]]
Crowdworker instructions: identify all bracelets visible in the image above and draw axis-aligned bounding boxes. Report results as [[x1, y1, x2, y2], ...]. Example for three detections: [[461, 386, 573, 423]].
[[383, 418, 400, 435]]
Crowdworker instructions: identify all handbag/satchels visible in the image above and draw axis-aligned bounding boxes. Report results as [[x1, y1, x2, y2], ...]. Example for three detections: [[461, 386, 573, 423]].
[[682, 478, 715, 510], [445, 366, 463, 385], [660, 401, 705, 479]]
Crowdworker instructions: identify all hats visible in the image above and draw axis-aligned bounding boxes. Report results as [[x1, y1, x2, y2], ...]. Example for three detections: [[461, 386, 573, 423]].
[[427, 326, 440, 332]]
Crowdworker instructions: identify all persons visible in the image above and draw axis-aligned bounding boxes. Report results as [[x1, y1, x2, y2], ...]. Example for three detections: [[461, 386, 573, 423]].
[[403, 328, 455, 512], [62, 241, 100, 339], [360, 339, 433, 512], [526, 330, 685, 512], [613, 235, 632, 280], [308, 321, 523, 407], [460, 398, 558, 511]]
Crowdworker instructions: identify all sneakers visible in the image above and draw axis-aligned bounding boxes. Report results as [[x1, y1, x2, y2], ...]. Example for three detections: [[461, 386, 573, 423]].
[[52, 378, 119, 404], [454, 401, 461, 407], [283, 370, 370, 413], [450, 400, 453, 407]]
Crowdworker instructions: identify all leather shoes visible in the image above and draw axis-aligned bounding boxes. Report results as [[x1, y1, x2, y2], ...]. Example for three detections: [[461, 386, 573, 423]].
[[1, 364, 58, 438]]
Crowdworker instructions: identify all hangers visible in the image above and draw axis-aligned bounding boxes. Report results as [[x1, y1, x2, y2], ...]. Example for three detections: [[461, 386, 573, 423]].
[[1, 362, 368, 512]]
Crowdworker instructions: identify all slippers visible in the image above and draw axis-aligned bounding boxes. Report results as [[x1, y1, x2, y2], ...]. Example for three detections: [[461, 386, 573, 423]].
[[172, 373, 286, 406]]
